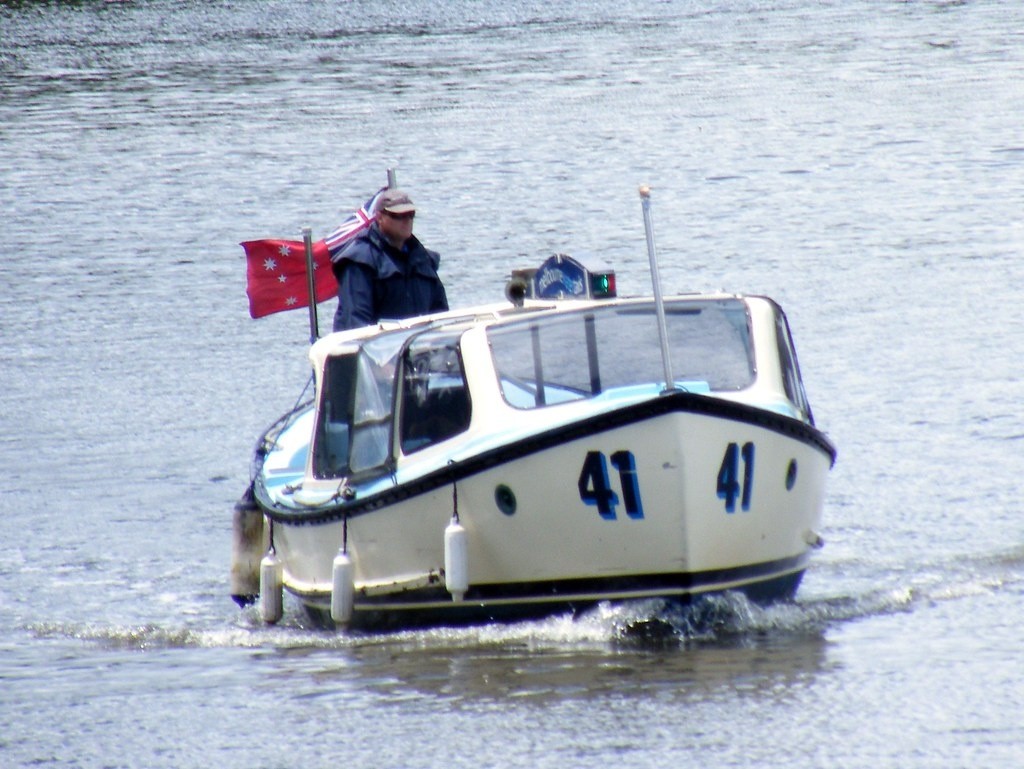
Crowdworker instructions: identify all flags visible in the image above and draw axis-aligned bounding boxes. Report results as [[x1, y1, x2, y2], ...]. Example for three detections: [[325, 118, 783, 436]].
[[240, 186, 391, 319]]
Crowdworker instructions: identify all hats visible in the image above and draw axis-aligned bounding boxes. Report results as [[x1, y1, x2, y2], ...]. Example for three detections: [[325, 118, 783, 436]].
[[375, 190, 416, 213]]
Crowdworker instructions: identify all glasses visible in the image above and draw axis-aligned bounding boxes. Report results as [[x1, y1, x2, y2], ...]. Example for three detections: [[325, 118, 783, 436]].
[[383, 210, 415, 221]]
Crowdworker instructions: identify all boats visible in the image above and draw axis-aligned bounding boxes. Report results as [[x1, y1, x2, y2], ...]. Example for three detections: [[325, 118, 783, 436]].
[[251, 163, 838, 646]]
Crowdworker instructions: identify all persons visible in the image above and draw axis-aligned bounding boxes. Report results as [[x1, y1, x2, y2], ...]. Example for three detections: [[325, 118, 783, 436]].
[[329, 190, 449, 424]]
